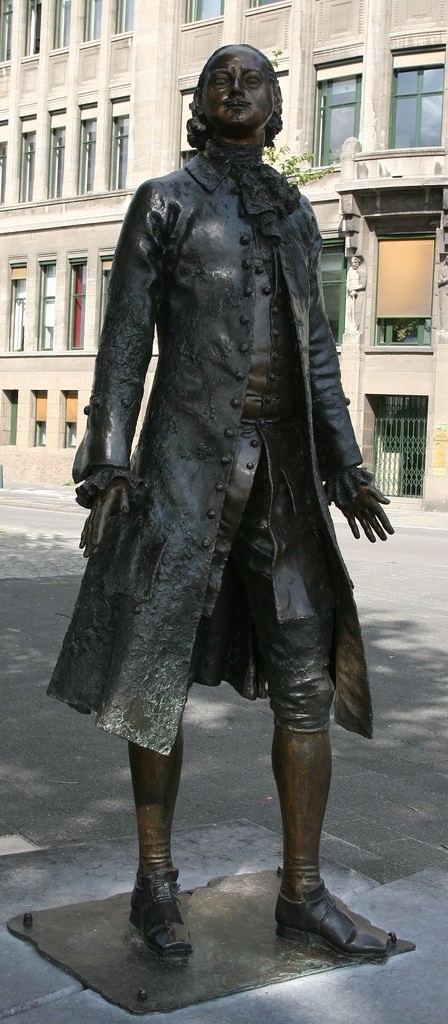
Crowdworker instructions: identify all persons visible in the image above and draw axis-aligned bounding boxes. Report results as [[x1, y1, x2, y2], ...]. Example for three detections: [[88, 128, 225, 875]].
[[48, 45, 395, 961]]
